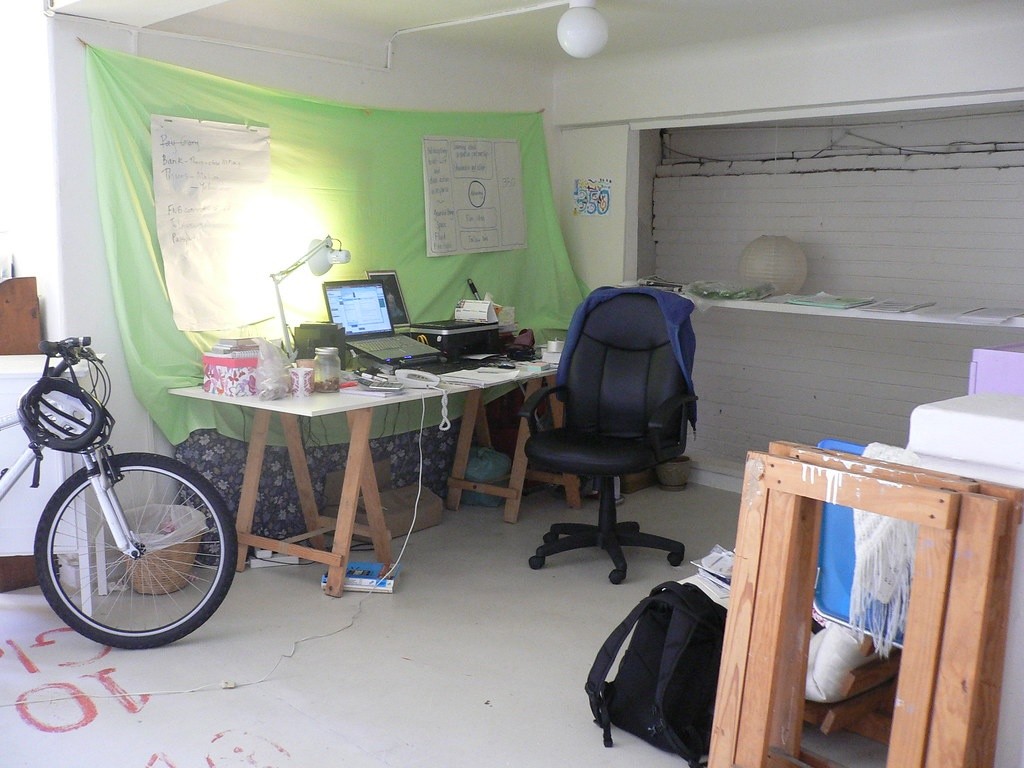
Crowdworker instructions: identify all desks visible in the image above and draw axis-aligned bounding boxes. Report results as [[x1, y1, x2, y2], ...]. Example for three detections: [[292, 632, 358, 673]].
[[167, 350, 583, 600], [0, 353, 106, 622]]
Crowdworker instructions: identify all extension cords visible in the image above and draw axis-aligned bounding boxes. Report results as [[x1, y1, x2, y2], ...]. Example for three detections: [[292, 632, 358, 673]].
[[249, 553, 299, 568]]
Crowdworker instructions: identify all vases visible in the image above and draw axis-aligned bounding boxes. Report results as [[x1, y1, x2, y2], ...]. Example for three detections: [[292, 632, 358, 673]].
[[656, 456, 691, 487]]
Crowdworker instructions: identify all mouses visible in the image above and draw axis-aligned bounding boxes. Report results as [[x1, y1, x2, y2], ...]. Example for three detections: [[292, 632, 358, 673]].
[[498, 361, 515, 369]]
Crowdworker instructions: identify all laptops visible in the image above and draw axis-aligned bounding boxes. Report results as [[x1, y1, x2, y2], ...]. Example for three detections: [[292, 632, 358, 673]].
[[322, 281, 441, 362]]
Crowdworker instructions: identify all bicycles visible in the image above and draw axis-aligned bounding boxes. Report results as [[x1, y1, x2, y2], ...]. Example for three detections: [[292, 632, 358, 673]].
[[0, 338, 238, 650]]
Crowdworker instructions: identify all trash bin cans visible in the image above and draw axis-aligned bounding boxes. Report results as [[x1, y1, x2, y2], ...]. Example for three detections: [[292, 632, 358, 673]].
[[122, 503, 208, 595]]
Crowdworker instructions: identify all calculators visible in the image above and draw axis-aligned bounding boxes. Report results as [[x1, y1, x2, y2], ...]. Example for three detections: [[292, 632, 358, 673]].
[[369, 382, 404, 392]]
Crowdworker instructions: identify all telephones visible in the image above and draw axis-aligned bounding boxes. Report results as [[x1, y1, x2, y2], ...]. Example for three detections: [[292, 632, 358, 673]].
[[395, 369, 441, 389]]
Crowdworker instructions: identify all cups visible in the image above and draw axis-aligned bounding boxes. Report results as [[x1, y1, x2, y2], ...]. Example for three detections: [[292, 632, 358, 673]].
[[289, 368, 315, 398]]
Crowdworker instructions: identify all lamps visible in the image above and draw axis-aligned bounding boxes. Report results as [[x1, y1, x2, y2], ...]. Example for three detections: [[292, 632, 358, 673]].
[[557, 0, 610, 59]]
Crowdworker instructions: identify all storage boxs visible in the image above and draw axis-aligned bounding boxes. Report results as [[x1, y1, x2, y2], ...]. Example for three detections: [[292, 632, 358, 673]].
[[203, 356, 260, 397], [410, 319, 500, 357]]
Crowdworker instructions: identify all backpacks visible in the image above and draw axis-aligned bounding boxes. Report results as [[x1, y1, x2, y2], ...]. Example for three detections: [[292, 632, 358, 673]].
[[583, 578, 729, 768]]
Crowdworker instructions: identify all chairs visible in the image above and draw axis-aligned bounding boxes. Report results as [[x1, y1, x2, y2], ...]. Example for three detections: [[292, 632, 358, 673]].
[[518, 285, 700, 584]]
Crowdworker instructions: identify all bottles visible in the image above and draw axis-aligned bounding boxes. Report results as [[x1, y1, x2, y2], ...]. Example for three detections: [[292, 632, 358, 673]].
[[313, 347, 340, 393], [159, 516, 175, 535]]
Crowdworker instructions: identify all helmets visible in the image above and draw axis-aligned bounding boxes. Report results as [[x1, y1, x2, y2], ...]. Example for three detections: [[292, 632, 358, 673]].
[[17, 377, 118, 456]]
